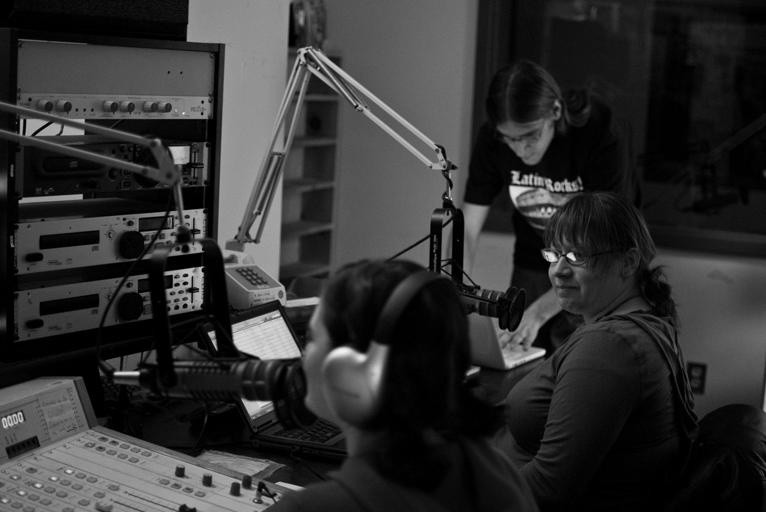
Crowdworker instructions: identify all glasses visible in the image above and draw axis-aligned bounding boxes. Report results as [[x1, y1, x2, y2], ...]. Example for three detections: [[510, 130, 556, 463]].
[[502, 119, 546, 144], [541, 248, 621, 266]]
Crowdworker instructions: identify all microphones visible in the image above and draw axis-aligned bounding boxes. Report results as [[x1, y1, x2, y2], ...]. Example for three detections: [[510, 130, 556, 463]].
[[453, 285, 528, 332], [109, 358, 307, 409]]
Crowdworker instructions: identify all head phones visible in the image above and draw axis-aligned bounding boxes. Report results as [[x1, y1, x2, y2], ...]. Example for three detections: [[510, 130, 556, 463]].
[[323, 268, 447, 423]]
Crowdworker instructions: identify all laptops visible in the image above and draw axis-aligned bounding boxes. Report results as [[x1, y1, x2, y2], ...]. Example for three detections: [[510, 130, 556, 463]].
[[200, 300, 346, 448], [466, 311, 546, 372]]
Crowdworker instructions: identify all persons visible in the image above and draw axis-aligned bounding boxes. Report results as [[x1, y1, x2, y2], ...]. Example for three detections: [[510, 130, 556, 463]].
[[446, 59, 627, 359], [259, 257, 540, 512], [495, 189, 699, 512]]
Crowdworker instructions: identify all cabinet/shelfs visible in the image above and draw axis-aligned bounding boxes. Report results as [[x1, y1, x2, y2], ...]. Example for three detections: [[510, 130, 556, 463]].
[[279, 50, 344, 283]]
